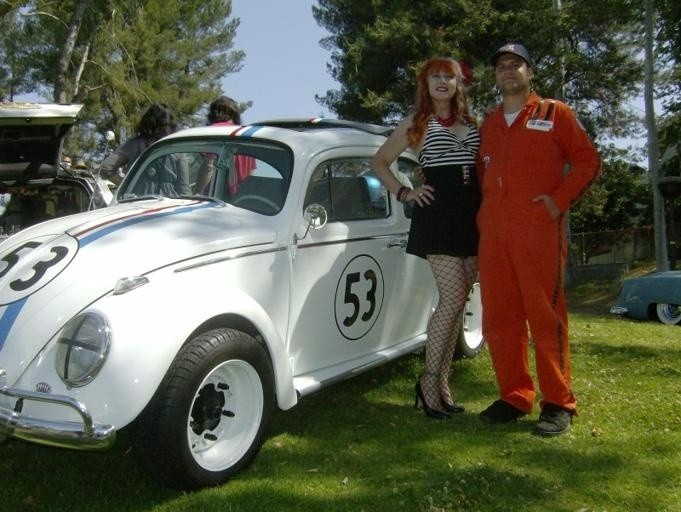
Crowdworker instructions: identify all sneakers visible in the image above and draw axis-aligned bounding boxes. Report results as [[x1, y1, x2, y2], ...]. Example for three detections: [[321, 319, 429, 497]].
[[536, 403, 572, 436], [479, 400, 530, 423]]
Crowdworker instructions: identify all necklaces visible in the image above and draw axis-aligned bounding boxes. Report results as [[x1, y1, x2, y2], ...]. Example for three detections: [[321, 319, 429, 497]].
[[435, 114, 457, 127]]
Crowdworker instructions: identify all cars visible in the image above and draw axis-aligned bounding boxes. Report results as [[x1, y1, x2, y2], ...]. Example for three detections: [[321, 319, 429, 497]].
[[0, 102, 114, 244]]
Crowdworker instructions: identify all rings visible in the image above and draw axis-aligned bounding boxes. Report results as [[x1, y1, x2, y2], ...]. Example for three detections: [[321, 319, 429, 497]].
[[419, 192, 423, 197], [421, 184, 425, 189]]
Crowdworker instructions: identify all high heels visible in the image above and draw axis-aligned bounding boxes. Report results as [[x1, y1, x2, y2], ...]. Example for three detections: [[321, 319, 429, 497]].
[[412, 382, 452, 422], [438, 385, 467, 415]]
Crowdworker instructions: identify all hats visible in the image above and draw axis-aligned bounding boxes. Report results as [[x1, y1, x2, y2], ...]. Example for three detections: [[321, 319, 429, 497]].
[[491, 42, 534, 67]]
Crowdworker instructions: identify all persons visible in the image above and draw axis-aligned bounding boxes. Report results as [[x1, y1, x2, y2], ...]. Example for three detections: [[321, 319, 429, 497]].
[[369, 56, 480, 419], [473, 44, 599, 436], [194, 96, 257, 203], [100, 101, 193, 198]]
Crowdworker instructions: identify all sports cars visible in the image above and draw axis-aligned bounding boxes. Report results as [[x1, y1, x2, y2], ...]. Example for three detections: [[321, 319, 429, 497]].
[[609, 270, 681, 325], [1, 118, 486, 493]]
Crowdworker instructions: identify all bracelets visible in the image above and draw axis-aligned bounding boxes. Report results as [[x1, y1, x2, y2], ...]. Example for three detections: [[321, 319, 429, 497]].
[[396, 186, 411, 202]]
